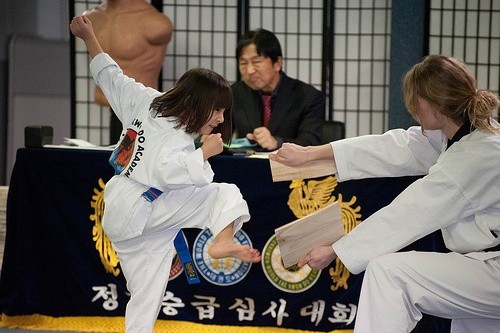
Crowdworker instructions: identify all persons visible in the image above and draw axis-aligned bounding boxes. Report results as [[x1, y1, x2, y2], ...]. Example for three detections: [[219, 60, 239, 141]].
[[205, 29, 326, 154], [77, 0, 173, 106], [70, 16, 263, 333], [269, 55, 500, 333]]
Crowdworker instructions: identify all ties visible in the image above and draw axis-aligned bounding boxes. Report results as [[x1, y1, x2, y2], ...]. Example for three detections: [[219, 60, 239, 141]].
[[261, 96, 272, 127]]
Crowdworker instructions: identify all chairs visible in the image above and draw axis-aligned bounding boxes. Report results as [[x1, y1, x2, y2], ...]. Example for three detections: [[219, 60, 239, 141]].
[[324, 120, 345, 144]]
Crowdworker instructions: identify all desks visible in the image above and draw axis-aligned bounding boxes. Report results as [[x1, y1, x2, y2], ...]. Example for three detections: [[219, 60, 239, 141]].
[[0, 147, 452, 333]]
